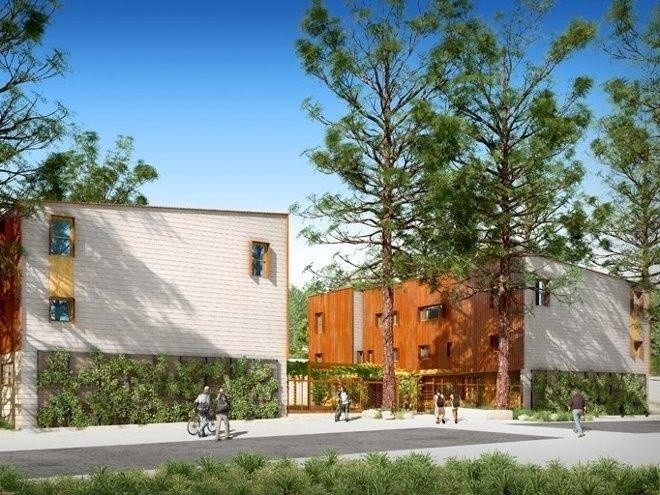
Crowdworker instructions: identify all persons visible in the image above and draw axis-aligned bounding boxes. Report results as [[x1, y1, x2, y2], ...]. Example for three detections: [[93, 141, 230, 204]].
[[213, 386, 233, 443], [432, 389, 446, 425], [448, 388, 465, 424], [568, 387, 586, 438], [402, 391, 412, 413], [194, 385, 211, 438], [332, 386, 349, 421]]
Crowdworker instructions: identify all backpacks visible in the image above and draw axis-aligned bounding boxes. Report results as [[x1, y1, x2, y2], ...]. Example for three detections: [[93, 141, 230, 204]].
[[437, 395, 444, 407], [453, 396, 460, 406]]
